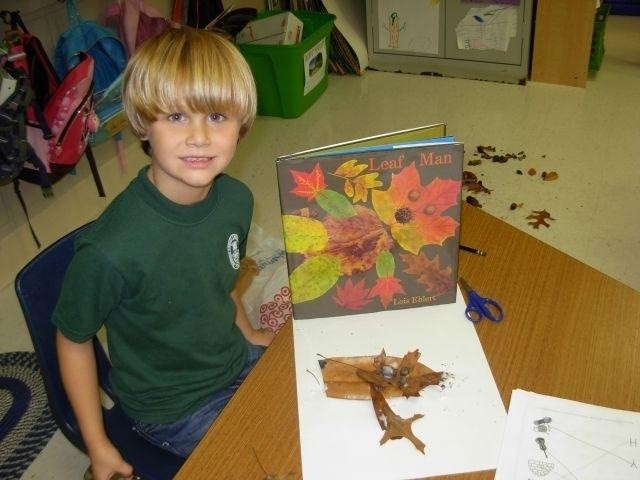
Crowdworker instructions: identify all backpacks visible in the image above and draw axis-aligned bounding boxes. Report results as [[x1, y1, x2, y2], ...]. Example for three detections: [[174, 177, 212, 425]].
[[0, 0, 172, 184]]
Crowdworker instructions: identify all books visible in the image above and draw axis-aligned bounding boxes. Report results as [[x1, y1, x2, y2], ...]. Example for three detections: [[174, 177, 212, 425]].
[[275, 120, 465, 321]]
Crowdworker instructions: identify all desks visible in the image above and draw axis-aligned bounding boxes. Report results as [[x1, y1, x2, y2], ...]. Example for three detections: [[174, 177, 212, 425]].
[[171, 199, 640, 480]]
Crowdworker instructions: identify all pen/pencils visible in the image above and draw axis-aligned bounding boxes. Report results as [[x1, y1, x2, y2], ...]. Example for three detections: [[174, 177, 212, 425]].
[[459, 243, 486, 256]]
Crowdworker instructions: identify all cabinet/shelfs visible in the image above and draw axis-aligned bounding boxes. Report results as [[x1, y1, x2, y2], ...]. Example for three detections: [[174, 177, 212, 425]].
[[364, 0, 533, 85]]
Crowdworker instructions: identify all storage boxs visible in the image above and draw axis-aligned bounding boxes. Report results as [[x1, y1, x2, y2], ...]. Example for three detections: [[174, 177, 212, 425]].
[[231, 7, 335, 122]]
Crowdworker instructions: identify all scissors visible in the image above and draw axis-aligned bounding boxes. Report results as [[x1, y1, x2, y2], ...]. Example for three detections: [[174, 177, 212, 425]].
[[458, 277, 504, 323]]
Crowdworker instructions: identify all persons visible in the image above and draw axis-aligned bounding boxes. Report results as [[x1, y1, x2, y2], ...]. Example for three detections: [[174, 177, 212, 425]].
[[50, 24, 270, 479]]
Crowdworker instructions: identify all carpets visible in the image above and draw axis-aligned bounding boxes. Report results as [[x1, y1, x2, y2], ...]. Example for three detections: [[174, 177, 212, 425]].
[[0, 351, 58, 479]]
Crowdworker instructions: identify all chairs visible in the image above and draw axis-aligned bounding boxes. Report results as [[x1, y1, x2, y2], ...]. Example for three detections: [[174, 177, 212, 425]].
[[13, 219, 186, 480]]
